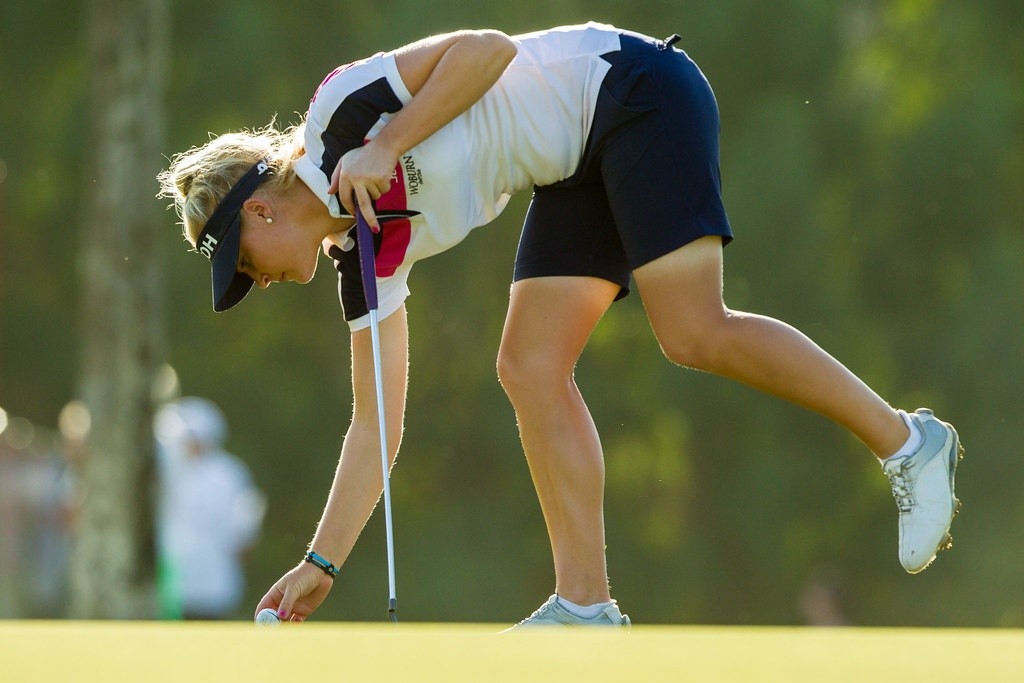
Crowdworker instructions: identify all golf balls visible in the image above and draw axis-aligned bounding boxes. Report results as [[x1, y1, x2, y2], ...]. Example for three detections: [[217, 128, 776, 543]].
[[256, 607, 282, 623]]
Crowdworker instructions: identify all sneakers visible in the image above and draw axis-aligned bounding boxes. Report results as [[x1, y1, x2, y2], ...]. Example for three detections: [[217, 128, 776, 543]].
[[502, 594, 639, 632], [883, 406, 965, 574]]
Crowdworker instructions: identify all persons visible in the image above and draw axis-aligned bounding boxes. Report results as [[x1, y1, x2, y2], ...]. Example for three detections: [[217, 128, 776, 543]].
[[154, 19, 962, 635], [156, 397, 269, 622]]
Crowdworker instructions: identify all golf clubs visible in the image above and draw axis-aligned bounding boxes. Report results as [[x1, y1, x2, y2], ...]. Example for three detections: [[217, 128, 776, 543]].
[[354, 201, 401, 623]]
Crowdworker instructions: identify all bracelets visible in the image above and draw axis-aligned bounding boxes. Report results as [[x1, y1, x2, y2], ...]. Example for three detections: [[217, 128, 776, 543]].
[[304, 549, 344, 585]]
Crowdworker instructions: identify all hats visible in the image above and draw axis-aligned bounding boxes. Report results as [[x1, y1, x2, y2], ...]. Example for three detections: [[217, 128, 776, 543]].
[[195, 160, 269, 312]]
